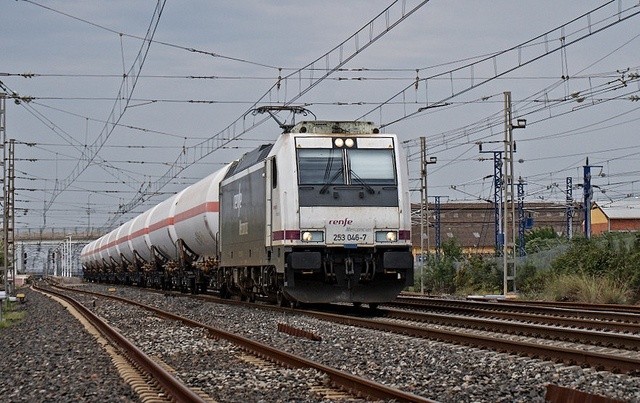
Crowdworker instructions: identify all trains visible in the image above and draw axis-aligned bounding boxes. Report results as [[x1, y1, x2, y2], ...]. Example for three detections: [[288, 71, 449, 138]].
[[80, 120, 414, 308]]
